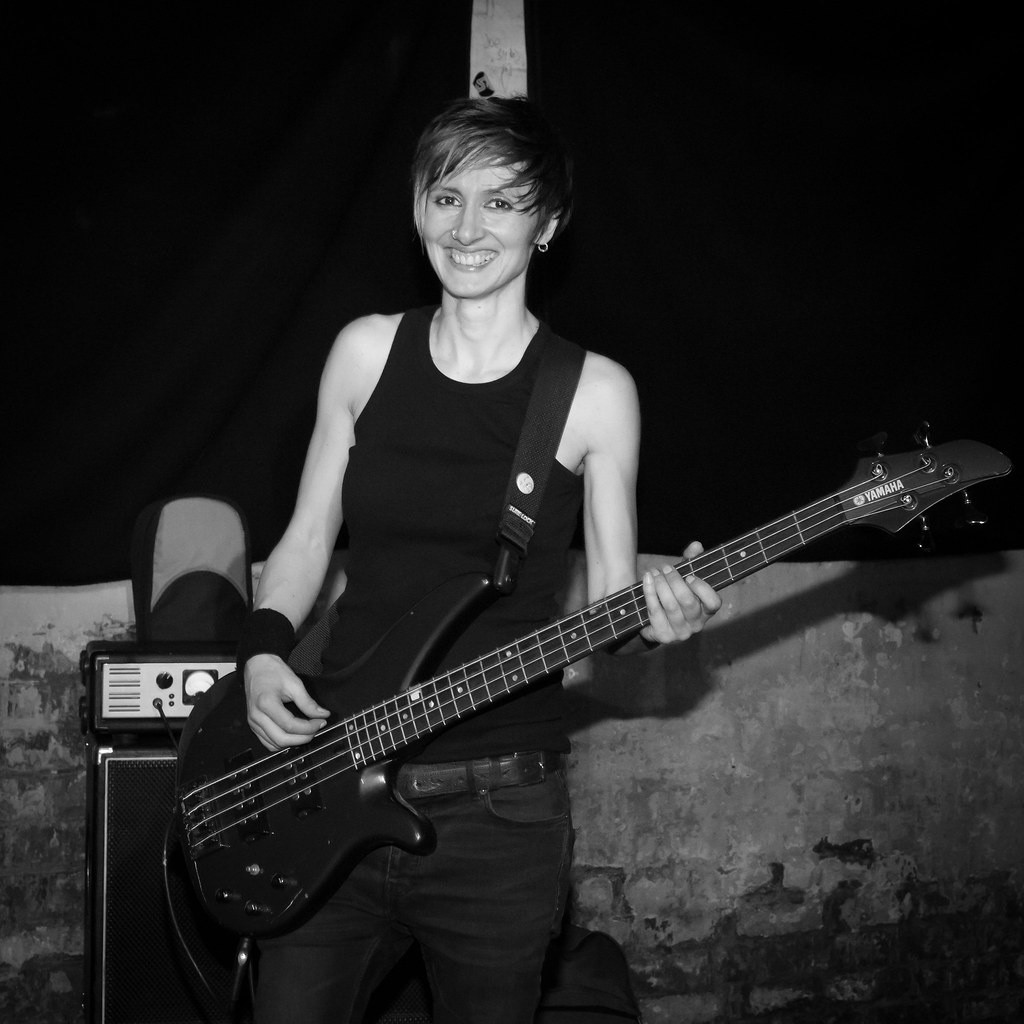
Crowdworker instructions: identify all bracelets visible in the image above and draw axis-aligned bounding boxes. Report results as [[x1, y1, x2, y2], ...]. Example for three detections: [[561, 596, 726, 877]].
[[240, 608, 294, 663]]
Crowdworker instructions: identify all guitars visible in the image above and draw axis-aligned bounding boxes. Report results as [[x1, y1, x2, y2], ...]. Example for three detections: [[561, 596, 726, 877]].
[[172, 417, 1018, 941]]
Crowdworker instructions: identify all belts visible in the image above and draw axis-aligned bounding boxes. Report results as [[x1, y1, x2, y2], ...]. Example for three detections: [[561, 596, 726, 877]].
[[395, 747, 564, 800]]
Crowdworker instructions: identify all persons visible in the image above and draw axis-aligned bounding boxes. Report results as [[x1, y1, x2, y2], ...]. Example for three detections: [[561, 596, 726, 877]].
[[238, 96, 721, 1024]]
